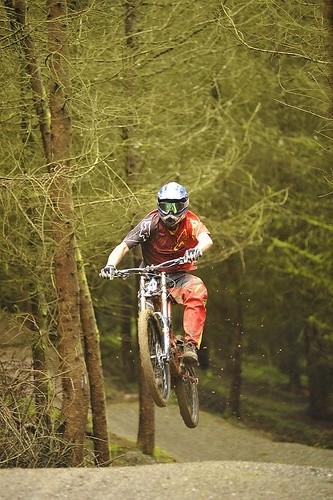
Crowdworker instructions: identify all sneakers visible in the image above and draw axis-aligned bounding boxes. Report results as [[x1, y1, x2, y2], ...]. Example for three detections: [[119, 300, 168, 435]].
[[153, 363, 163, 387], [182, 342, 198, 367]]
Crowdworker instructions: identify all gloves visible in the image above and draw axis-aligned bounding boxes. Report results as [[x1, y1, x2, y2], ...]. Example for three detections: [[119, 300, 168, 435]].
[[184, 248, 203, 265], [100, 265, 118, 281]]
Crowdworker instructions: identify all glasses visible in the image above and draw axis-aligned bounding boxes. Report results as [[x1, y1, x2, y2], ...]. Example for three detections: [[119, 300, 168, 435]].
[[157, 201, 188, 214]]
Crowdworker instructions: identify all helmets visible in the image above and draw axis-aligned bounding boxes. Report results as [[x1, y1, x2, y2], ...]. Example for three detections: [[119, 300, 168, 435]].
[[156, 182, 189, 228]]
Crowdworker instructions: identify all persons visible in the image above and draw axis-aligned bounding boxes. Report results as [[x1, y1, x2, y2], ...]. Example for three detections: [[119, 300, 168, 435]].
[[101, 182, 213, 367]]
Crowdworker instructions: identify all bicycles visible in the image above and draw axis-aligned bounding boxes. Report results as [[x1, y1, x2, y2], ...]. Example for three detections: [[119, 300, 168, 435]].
[[98, 249, 203, 428]]
